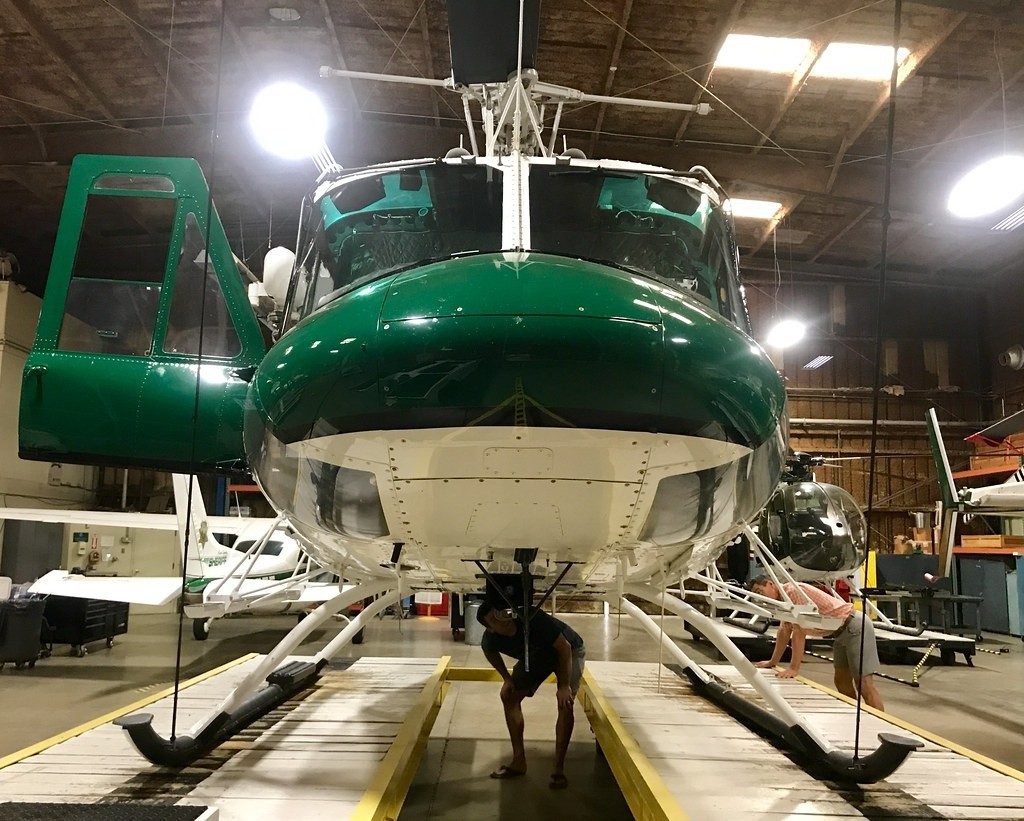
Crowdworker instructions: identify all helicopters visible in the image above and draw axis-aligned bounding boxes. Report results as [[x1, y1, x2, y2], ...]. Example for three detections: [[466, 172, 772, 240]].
[[0, 0, 1023, 821]]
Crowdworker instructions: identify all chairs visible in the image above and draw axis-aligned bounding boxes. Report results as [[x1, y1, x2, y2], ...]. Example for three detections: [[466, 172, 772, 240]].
[[596, 232, 680, 280], [350, 231, 441, 286]]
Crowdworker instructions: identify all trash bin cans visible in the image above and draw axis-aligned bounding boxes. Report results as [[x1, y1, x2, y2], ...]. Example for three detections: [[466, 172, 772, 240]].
[[40, 575, 130, 657], [0, 599, 46, 670]]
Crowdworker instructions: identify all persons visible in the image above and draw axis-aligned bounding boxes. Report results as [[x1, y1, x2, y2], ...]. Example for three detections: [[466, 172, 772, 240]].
[[749, 575, 884, 712], [477, 599, 585, 788]]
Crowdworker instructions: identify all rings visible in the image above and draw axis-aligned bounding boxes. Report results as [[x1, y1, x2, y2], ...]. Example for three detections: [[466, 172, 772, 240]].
[[568, 701, 570, 703]]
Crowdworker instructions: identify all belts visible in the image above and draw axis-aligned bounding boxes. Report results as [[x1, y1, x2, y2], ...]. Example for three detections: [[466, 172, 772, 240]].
[[831, 608, 856, 638]]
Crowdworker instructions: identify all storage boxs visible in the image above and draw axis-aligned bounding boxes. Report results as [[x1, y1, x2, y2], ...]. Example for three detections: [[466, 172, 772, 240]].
[[969, 455, 1022, 471], [961, 534, 1024, 549]]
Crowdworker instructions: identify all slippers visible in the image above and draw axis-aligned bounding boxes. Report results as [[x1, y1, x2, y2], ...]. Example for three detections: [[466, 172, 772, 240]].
[[549, 773, 568, 788], [489, 765, 526, 778]]
